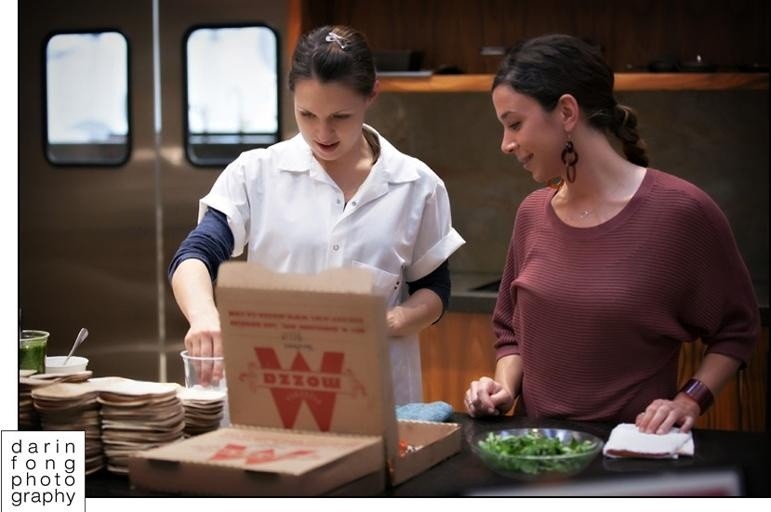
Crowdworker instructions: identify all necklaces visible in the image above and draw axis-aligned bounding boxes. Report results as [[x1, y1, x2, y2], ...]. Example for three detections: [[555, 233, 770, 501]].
[[576, 182, 619, 219]]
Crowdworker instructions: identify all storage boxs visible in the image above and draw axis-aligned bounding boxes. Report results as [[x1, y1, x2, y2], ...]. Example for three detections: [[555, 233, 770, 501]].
[[128, 260, 464, 496]]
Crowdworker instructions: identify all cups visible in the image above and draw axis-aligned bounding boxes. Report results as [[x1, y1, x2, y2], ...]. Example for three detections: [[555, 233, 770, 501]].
[[180, 349, 230, 429], [19, 330, 89, 374]]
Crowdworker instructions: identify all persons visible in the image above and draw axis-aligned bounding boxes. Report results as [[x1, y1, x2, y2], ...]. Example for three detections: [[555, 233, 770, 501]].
[[464, 34, 762, 436], [167, 27, 466, 407]]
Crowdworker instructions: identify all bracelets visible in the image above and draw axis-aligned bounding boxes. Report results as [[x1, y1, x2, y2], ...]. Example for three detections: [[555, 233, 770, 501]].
[[678, 380, 717, 416]]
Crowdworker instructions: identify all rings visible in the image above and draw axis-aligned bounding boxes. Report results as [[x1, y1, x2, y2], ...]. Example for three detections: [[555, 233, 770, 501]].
[[468, 400, 473, 408]]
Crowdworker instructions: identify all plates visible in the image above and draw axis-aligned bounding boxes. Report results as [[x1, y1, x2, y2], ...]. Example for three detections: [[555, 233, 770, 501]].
[[468, 427, 604, 482]]
